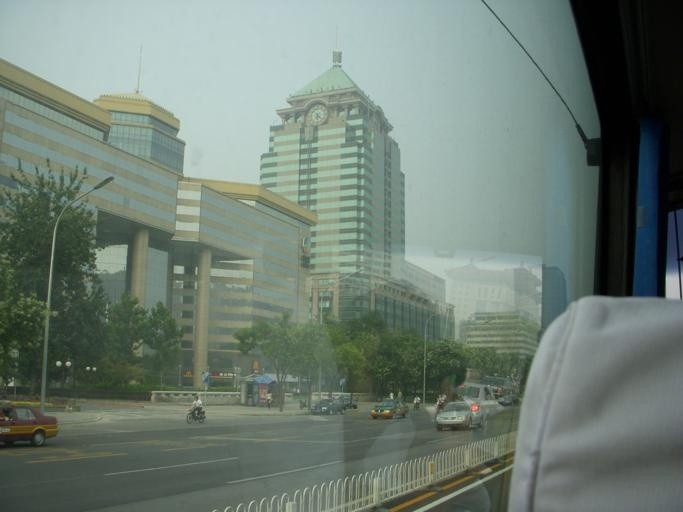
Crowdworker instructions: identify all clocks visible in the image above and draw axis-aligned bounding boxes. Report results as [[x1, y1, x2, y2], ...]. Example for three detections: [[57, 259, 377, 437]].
[[305, 104, 328, 126]]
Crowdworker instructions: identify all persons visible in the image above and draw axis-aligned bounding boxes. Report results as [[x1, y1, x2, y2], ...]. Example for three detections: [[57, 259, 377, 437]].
[[266, 394, 271, 408], [413, 395, 419, 407], [191, 394, 201, 415]]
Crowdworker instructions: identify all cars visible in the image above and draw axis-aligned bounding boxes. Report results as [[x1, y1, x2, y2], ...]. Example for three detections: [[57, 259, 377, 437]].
[[466, 400, 490, 430], [334, 396, 358, 409], [1, 399, 61, 447], [311, 398, 346, 415], [435, 401, 472, 431], [370, 398, 409, 419]]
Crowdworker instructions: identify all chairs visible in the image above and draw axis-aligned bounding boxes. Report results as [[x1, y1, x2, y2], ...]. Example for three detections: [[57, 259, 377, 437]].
[[507, 294, 683, 511]]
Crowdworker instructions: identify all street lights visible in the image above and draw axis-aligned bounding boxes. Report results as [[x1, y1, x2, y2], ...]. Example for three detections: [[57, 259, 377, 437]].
[[11, 348, 21, 399], [40, 174, 116, 414], [56, 360, 72, 394]]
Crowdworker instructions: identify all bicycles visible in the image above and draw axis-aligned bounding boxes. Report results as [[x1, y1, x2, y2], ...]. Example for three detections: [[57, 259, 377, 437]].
[[186, 406, 206, 424]]
